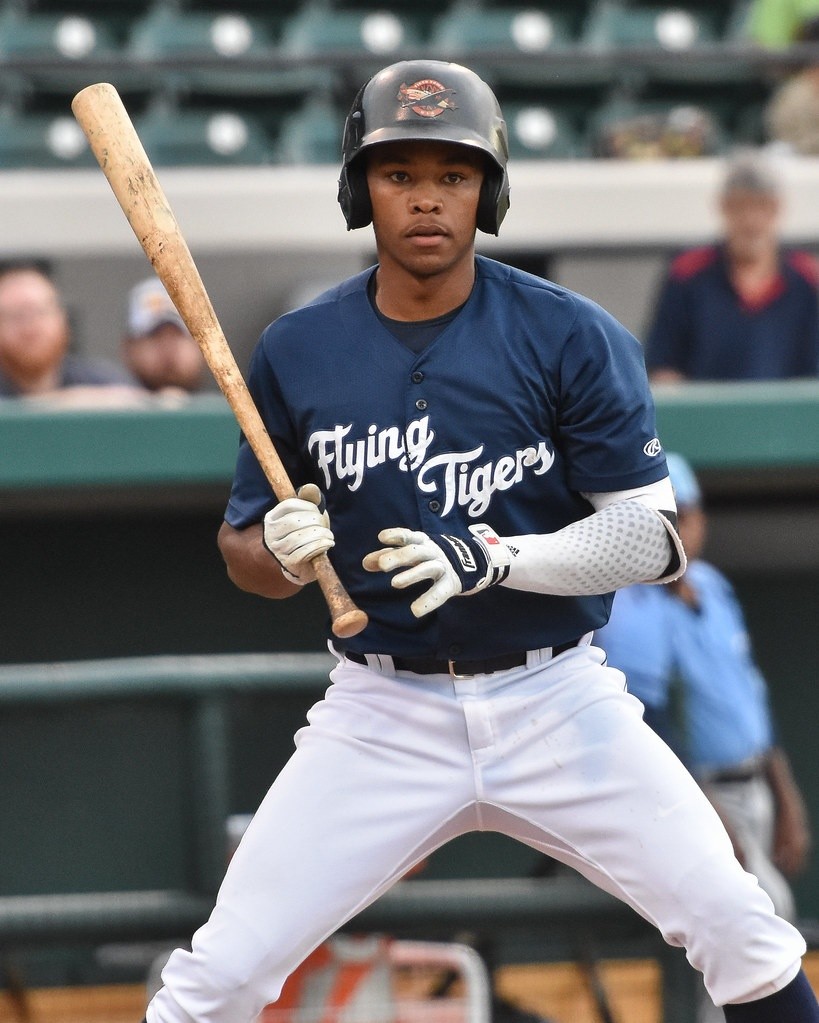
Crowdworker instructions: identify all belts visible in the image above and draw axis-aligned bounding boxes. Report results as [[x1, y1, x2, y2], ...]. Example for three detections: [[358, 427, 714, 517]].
[[344, 638, 579, 680], [710, 768, 758, 785]]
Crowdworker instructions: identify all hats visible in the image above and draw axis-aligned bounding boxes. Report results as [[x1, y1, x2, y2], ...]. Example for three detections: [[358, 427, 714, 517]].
[[665, 451, 700, 508], [125, 276, 191, 338]]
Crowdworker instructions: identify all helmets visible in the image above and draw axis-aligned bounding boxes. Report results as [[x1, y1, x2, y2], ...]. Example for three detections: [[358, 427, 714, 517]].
[[336, 59, 510, 237]]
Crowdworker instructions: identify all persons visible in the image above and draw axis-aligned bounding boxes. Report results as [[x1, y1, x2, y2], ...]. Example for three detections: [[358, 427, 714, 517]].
[[0, 252, 213, 419], [640, 151, 817, 390], [140, 58, 818, 1023], [589, 448, 809, 1023]]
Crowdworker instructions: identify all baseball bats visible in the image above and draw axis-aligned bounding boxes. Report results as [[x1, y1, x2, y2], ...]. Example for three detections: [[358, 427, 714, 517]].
[[70, 82, 370, 639]]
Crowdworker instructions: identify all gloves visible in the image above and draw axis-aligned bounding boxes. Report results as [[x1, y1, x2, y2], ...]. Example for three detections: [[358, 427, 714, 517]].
[[262, 483, 335, 586], [360, 522, 512, 618]]
